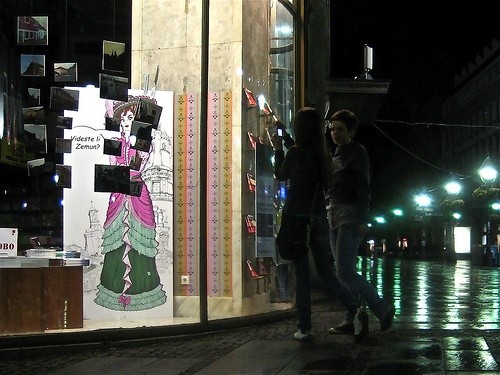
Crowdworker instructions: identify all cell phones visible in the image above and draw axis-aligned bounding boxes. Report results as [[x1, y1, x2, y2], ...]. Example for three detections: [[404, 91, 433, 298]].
[[276, 128, 283, 137]]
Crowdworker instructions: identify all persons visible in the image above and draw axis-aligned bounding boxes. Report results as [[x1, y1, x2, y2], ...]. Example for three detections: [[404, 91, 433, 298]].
[[271, 107, 369, 342], [329, 109, 395, 334]]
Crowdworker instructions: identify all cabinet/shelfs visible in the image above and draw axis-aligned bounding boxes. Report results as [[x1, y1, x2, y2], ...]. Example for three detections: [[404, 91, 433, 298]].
[[0, 258, 90, 334]]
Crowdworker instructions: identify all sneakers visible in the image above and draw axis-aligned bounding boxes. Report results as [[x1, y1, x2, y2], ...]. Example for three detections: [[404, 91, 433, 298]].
[[328, 320, 355, 335], [352, 308, 370, 344], [294, 328, 314, 342], [379, 306, 395, 335]]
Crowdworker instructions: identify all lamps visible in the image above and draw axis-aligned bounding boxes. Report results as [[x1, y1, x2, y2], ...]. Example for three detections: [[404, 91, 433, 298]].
[[360, 43, 373, 81]]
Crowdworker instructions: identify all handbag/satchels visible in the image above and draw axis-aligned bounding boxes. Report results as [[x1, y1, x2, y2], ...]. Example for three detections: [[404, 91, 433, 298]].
[[275, 214, 313, 261]]
